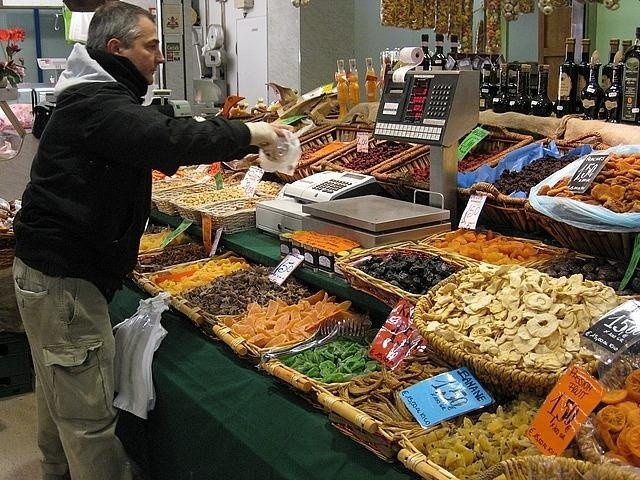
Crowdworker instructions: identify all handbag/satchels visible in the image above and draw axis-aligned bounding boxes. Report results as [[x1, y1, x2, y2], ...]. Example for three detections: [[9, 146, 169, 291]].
[[29, 88, 54, 139]]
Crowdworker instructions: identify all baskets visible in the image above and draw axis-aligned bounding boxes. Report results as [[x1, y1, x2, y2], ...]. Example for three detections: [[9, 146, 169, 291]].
[[336, 124, 640, 480], [128, 223, 337, 431], [1, 232, 16, 269], [131, 110, 637, 479], [146, 152, 290, 235], [231, 105, 336, 182]]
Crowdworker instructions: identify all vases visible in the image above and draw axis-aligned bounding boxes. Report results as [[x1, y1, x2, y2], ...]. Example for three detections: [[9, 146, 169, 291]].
[[0, 76, 8, 89]]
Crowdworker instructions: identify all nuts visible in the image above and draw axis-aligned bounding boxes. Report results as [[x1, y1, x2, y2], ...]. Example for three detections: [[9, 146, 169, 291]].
[[537, 152, 640, 214], [403, 142, 513, 184], [136, 227, 640, 480], [341, 139, 416, 172], [482, 153, 580, 197], [379, 0, 620, 55], [149, 161, 284, 216], [296, 143, 328, 163]]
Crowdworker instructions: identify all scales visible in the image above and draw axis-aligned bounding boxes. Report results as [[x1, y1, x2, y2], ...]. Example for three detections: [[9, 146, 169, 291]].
[[301, 69, 480, 249]]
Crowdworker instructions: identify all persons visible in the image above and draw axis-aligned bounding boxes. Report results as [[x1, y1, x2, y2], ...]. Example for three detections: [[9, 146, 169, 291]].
[[11, 1, 293, 480]]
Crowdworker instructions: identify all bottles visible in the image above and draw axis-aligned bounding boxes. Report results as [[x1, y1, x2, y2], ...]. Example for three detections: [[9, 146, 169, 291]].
[[419, 33, 432, 72], [431, 33, 449, 71], [448, 34, 460, 54], [347, 59, 359, 108], [380, 46, 401, 68], [479, 25, 640, 125], [364, 56, 377, 103], [335, 58, 347, 122]]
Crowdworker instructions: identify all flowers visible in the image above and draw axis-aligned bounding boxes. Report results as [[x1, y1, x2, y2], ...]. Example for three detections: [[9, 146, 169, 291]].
[[0, 23, 27, 88]]
